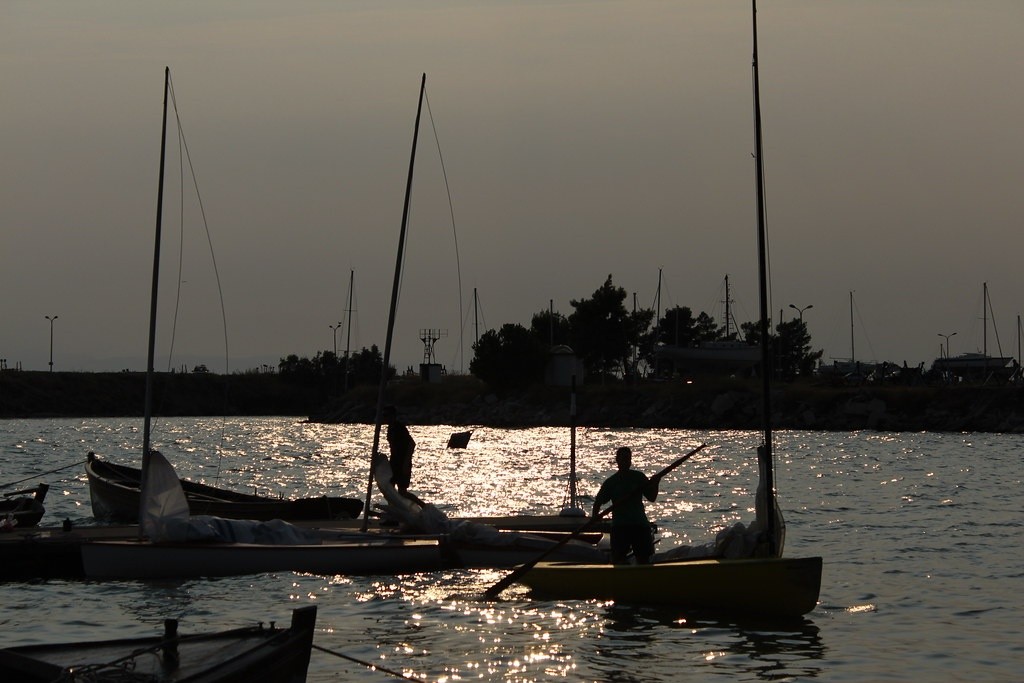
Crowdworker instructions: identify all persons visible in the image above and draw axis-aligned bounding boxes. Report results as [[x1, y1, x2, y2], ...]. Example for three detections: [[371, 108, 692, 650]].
[[378, 404, 426, 526], [594, 448, 661, 564]]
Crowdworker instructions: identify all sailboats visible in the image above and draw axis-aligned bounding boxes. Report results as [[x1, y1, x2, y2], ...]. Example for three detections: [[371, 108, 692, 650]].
[[830, 291, 925, 389], [514, 1, 826, 624], [1, 71, 658, 585], [653, 273, 762, 362], [936, 280, 1014, 369]]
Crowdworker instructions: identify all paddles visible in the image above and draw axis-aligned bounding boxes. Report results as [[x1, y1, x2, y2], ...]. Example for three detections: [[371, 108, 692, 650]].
[[475, 443, 707, 605]]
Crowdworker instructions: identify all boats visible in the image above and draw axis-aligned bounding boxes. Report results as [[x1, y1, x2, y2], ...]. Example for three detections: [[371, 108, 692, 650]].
[[1, 600, 319, 683]]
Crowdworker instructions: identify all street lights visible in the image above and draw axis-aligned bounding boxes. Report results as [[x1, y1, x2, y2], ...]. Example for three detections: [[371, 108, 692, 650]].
[[44, 315, 59, 372], [789, 304, 814, 325], [329, 324, 342, 358], [937, 332, 957, 358]]
